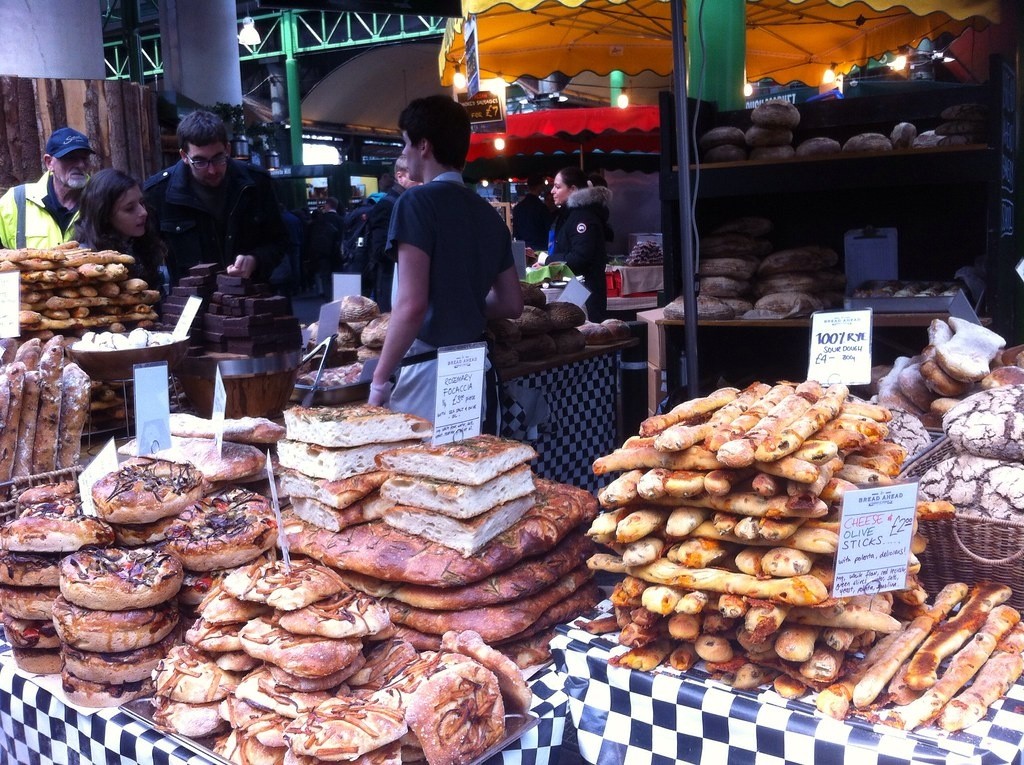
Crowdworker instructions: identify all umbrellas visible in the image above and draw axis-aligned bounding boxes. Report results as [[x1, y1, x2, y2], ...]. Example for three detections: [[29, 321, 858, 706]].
[[438, 0, 1002, 86]]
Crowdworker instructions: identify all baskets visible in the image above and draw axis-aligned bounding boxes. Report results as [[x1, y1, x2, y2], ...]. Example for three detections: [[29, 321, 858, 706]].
[[895, 437, 1024, 621]]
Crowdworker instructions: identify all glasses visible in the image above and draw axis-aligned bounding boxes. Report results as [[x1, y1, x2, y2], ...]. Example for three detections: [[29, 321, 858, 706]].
[[185, 149, 227, 170]]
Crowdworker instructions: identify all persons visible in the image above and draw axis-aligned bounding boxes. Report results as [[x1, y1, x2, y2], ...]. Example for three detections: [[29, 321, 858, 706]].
[[369, 94, 524, 441], [538, 165, 613, 323], [277, 195, 304, 296], [365, 155, 423, 314], [343, 172, 395, 274], [70, 168, 166, 317], [513, 171, 553, 251], [586, 174, 615, 243], [0, 128, 92, 250], [131, 111, 290, 322], [307, 196, 344, 298]]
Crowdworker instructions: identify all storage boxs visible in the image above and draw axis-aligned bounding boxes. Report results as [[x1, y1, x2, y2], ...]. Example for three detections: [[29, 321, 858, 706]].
[[628, 231, 662, 254], [648, 361, 669, 413], [636, 307, 665, 369], [843, 279, 969, 312]]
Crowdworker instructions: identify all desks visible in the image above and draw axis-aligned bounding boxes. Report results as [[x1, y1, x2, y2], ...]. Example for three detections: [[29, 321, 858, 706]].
[[0, 665, 589, 765], [498, 337, 643, 454], [547, 597, 1024, 765]]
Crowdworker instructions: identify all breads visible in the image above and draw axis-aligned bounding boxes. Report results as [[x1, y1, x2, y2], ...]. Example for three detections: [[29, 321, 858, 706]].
[[0, 100, 1024, 764]]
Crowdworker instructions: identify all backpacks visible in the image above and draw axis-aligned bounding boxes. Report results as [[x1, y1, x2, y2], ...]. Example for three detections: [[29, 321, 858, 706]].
[[343, 196, 397, 276]]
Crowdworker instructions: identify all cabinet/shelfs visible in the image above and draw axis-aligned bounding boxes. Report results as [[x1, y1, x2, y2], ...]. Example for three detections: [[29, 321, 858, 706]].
[[657, 126, 995, 326]]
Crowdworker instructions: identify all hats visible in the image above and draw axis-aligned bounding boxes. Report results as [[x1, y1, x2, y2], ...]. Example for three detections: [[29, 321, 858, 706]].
[[46, 127, 97, 158]]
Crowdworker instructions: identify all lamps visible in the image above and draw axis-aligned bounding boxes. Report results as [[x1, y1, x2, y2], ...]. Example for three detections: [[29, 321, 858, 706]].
[[264, 150, 280, 169], [238, 4, 262, 45], [230, 134, 249, 160]]
